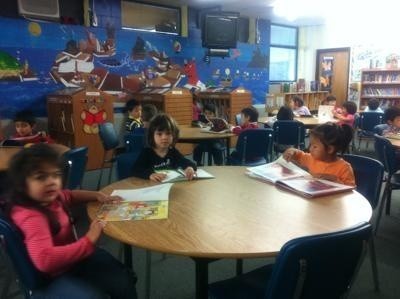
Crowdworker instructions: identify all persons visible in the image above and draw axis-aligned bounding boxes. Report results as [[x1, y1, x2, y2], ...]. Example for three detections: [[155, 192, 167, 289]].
[[283, 122, 356, 190], [274, 105, 297, 153], [291, 95, 312, 118], [225, 107, 262, 166], [365, 98, 385, 113], [134, 113, 198, 182], [1, 141, 138, 299], [321, 95, 342, 115], [382, 106, 400, 138], [130, 103, 158, 146], [191, 104, 223, 165], [334, 101, 356, 127], [1, 109, 54, 148], [118, 97, 143, 146]]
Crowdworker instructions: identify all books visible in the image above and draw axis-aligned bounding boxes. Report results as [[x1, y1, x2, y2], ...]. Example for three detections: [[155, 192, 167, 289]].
[[92, 182, 174, 222], [363, 71, 399, 106], [154, 165, 215, 183], [243, 153, 357, 198]]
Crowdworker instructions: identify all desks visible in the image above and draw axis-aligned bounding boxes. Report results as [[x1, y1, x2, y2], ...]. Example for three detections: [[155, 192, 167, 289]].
[[3, 140, 69, 168], [92, 167, 375, 298]]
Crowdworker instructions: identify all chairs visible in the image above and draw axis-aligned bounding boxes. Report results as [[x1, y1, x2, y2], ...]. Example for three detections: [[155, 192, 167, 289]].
[[2, 202, 139, 298], [206, 220, 372, 298], [336, 154, 383, 289], [54, 110, 399, 259]]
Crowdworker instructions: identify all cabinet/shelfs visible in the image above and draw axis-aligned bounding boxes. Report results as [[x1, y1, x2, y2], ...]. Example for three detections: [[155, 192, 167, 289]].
[[194, 92, 252, 150], [132, 92, 195, 156], [356, 67, 400, 115], [265, 91, 329, 114], [46, 83, 114, 171]]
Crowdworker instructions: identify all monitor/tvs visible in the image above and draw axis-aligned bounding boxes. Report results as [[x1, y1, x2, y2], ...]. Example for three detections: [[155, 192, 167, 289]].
[[201, 12, 240, 48]]
